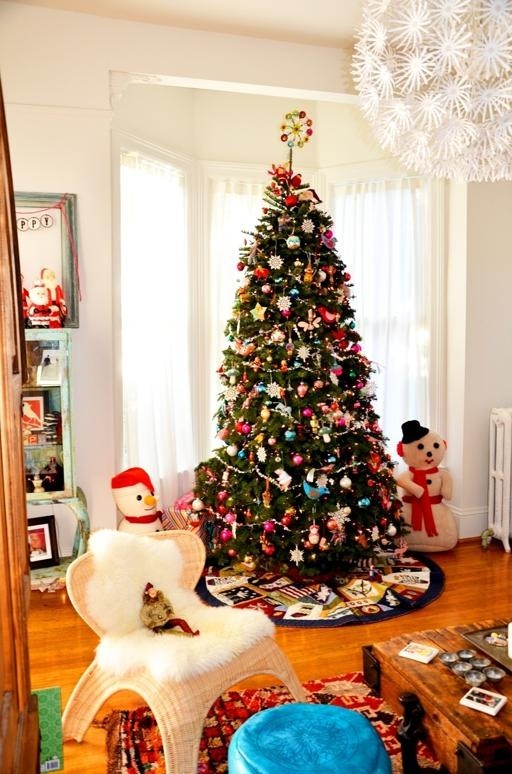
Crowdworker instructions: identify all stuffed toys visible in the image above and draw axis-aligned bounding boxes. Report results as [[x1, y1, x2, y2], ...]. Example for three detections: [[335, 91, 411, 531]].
[[111, 467, 165, 532], [396, 420, 459, 553]]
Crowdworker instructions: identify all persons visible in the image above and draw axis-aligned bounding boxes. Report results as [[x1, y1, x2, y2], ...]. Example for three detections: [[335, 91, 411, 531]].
[[20, 267, 67, 329], [140, 583, 200, 637]]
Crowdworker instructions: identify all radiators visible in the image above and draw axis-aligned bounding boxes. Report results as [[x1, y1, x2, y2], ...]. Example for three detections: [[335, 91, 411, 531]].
[[480, 406, 512, 552]]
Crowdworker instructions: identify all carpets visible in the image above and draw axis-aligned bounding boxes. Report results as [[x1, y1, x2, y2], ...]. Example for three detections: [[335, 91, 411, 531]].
[[196, 551, 446, 628], [94, 668, 449, 773]]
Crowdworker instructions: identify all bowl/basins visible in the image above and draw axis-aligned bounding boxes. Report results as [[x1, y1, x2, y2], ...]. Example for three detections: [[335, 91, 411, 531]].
[[437, 648, 506, 688]]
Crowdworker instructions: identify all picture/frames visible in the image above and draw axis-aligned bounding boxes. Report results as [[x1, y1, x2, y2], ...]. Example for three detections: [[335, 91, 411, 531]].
[[18, 388, 53, 444], [14, 190, 80, 328], [27, 514, 61, 571]]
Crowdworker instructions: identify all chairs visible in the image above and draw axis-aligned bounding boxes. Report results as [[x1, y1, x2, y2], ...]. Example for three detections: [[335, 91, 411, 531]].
[[56, 527, 310, 774]]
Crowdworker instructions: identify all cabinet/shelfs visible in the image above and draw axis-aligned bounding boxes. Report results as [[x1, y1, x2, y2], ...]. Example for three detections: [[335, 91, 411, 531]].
[[0, 79, 43, 773], [21, 329, 74, 503]]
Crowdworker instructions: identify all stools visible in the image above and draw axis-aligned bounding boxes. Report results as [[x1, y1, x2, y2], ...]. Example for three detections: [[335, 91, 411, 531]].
[[224, 702, 394, 774]]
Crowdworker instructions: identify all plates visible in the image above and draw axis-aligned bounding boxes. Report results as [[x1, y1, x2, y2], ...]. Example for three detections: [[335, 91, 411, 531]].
[[484, 632, 507, 648]]
